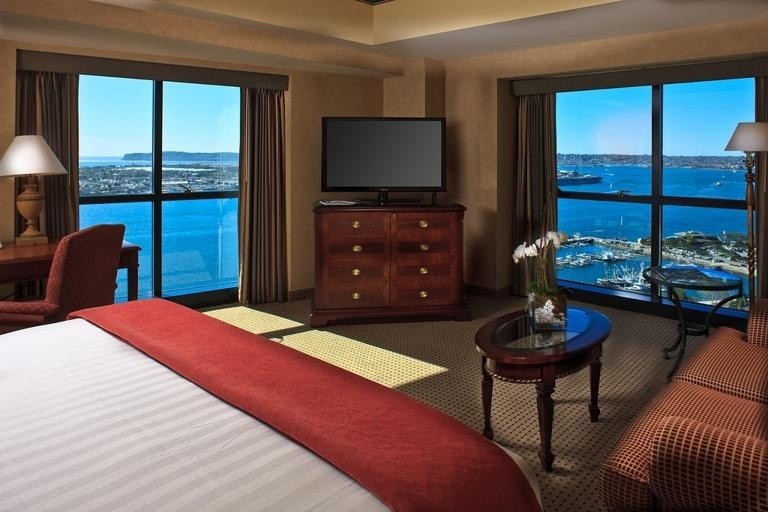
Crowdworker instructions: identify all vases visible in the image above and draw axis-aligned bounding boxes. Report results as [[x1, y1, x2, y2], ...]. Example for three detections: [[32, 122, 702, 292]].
[[531, 293, 569, 333]]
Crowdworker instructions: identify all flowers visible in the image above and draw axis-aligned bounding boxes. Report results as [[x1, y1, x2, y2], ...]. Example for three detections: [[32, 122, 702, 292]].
[[513, 230, 567, 294]]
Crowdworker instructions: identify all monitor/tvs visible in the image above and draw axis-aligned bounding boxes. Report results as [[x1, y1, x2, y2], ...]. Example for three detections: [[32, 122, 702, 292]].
[[321, 115, 447, 207]]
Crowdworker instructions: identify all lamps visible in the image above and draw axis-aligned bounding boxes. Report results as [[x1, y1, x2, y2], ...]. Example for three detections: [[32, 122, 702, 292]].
[[725, 122, 768, 306], [0, 134, 68, 248]]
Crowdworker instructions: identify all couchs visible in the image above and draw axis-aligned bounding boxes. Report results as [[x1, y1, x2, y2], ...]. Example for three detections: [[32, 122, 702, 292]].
[[601, 300, 768, 512]]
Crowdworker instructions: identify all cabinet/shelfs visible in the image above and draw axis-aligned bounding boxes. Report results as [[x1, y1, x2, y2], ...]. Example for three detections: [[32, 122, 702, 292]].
[[310, 201, 467, 327]]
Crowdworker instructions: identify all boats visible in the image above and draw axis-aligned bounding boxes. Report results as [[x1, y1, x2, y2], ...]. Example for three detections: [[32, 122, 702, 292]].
[[596, 262, 699, 291], [556, 249, 640, 270]]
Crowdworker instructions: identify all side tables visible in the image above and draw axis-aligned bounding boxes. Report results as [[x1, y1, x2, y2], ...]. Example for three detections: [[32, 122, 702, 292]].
[[643, 267, 743, 383]]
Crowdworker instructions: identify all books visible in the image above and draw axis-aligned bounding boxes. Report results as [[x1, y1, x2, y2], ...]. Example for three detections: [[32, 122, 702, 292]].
[[320, 200, 357, 207]]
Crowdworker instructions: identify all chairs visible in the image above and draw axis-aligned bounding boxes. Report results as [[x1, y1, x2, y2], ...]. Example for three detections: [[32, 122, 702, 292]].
[[0, 224, 125, 335]]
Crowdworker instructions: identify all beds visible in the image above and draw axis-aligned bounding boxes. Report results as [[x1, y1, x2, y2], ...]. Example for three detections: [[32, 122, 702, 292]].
[[0, 299, 546, 512]]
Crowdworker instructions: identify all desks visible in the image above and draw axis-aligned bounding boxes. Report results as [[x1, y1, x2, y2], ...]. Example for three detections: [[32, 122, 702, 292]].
[[0, 232, 141, 301]]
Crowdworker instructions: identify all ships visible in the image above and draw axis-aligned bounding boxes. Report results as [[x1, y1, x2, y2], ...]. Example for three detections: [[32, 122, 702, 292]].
[[557, 169, 603, 187]]
